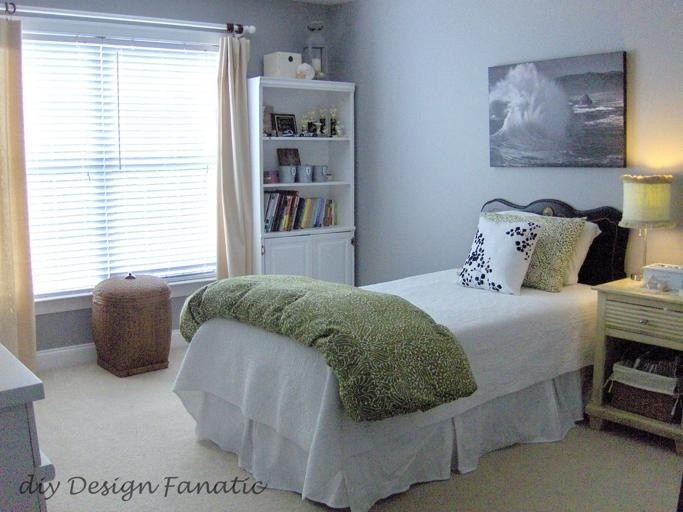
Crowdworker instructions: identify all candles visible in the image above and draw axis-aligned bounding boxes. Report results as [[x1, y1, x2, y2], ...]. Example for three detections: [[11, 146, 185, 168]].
[[312, 58, 321, 72]]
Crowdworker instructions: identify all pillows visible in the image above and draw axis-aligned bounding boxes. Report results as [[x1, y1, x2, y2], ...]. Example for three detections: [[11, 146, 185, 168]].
[[457, 210, 601, 295]]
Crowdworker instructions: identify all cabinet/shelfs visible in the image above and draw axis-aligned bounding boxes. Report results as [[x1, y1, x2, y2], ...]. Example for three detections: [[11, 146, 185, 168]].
[[248, 77, 356, 285], [1, 344, 56, 511]]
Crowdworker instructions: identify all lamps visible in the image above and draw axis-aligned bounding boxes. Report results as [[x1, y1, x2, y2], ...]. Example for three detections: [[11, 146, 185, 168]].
[[617, 174, 676, 281]]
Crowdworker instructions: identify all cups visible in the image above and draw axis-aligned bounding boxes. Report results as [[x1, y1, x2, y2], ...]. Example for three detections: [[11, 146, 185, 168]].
[[278, 164, 330, 183]]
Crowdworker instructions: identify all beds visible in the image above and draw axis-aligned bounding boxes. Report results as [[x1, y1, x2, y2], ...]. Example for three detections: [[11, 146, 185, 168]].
[[201, 199, 630, 512]]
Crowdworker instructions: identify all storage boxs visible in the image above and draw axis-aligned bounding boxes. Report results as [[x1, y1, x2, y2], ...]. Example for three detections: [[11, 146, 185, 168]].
[[90, 274, 172, 378]]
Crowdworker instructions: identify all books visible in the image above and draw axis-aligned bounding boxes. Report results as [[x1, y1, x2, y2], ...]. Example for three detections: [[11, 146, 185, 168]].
[[623, 348, 683, 377], [264, 189, 336, 234]]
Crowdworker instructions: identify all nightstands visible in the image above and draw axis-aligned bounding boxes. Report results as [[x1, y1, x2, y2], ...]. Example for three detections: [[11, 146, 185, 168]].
[[584, 276, 683, 454]]
